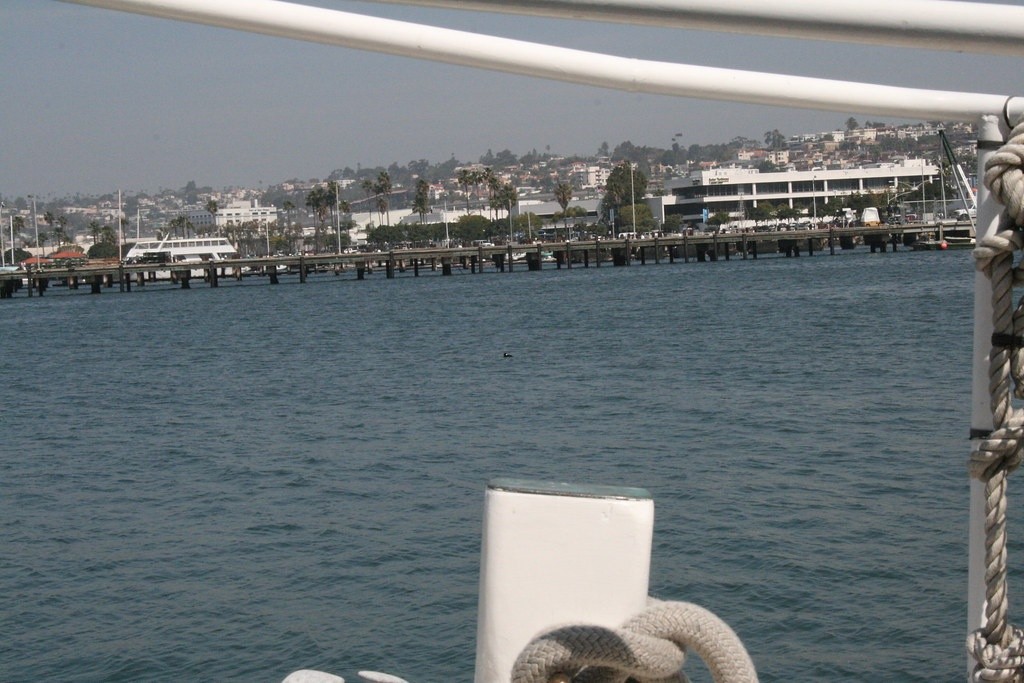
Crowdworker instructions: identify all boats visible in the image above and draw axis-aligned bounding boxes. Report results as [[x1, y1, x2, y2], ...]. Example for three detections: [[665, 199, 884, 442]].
[[120, 236, 238, 283]]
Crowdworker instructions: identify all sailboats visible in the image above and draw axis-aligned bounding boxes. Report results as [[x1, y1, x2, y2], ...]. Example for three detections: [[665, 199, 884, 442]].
[[912, 128, 979, 251]]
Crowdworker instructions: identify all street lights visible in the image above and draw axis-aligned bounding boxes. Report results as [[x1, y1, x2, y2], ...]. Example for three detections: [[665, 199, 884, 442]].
[[630, 163, 638, 239]]
